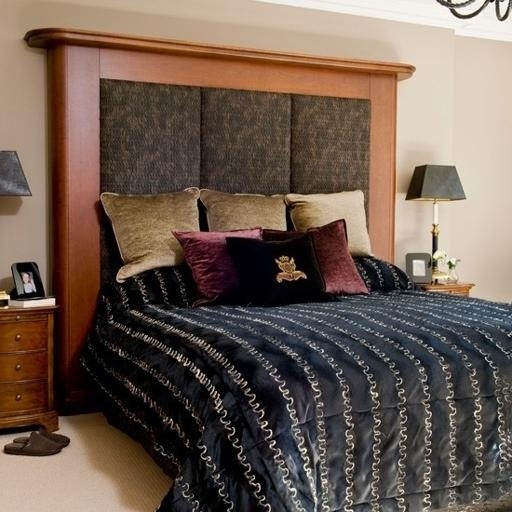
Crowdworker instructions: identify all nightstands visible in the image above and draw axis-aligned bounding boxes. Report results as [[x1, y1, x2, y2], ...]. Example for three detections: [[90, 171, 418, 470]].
[[1, 303, 62, 433], [416, 281, 474, 299]]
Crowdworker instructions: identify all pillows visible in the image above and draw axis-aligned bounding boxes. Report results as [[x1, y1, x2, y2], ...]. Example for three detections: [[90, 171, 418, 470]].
[[100, 187, 421, 312]]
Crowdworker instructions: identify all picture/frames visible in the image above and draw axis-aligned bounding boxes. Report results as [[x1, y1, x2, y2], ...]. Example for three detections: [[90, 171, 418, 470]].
[[405, 252, 433, 285], [10, 262, 46, 300]]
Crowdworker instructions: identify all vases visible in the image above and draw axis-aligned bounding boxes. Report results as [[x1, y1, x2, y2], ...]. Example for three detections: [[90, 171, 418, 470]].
[[446, 265, 459, 284]]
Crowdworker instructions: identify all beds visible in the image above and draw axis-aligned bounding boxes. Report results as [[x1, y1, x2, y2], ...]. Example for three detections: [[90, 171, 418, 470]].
[[24, 26, 512, 512]]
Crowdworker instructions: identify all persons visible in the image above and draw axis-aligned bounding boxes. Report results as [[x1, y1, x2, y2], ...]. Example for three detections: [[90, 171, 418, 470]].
[[22, 273, 36, 293]]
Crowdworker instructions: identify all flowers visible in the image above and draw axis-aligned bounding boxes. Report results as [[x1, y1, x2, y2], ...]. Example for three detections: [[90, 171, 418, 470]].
[[433, 250, 461, 270]]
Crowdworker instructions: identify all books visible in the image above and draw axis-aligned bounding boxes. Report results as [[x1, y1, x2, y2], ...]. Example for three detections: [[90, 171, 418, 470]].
[[8, 295, 57, 308]]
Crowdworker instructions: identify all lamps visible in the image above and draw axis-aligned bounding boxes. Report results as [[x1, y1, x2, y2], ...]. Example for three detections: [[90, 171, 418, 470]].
[[0, 150, 33, 197], [404, 164, 467, 285]]
